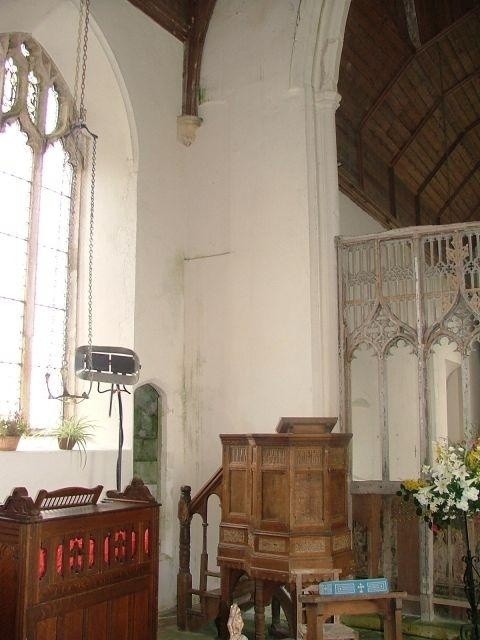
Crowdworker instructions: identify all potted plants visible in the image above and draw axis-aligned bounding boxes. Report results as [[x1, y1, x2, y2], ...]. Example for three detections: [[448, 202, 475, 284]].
[[0, 398, 27, 450], [31, 413, 102, 471]]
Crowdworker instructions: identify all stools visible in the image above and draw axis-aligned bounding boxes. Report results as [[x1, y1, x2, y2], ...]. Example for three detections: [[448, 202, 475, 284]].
[[298, 591, 407, 640]]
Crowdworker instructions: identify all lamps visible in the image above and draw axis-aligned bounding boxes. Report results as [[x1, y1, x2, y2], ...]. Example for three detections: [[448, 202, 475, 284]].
[[44, 0, 98, 401]]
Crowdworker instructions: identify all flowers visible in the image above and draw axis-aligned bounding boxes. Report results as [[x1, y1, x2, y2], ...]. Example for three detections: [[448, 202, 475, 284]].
[[397, 434, 480, 540]]
